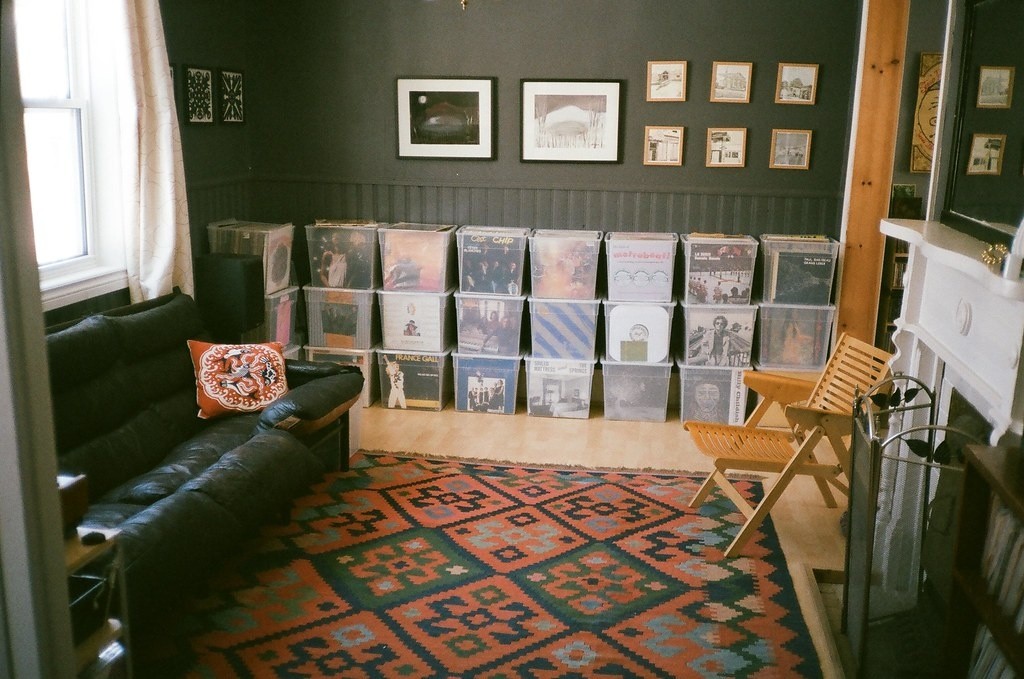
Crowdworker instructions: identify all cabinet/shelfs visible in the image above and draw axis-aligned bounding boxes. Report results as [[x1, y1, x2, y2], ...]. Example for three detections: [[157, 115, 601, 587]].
[[872, 236, 909, 378], [61, 526, 135, 679], [939, 444, 1024, 679]]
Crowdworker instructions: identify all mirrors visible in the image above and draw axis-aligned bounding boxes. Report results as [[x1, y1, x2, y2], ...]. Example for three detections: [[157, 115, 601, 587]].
[[941, 0, 1024, 260]]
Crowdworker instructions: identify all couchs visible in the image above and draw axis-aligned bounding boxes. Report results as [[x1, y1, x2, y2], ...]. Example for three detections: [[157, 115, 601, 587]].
[[43, 284, 366, 640]]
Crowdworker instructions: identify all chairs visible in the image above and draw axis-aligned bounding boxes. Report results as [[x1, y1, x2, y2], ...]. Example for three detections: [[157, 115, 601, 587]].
[[686, 331, 897, 560]]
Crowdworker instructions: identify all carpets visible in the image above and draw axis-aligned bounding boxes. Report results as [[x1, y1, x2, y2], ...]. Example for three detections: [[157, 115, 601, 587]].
[[153, 448, 827, 679]]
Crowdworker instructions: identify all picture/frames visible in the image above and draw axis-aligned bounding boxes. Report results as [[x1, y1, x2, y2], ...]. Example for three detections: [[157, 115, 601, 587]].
[[519, 78, 623, 165], [393, 74, 498, 162], [909, 52, 945, 174], [975, 66, 1016, 109], [965, 132, 1007, 175], [168, 62, 248, 127], [643, 59, 818, 171]]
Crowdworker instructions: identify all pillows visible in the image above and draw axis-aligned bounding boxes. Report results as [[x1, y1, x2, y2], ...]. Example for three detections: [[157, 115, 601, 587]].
[[186, 337, 290, 420]]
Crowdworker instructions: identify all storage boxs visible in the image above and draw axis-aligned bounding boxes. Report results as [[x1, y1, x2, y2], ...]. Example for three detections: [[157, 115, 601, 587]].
[[205, 216, 840, 428]]
